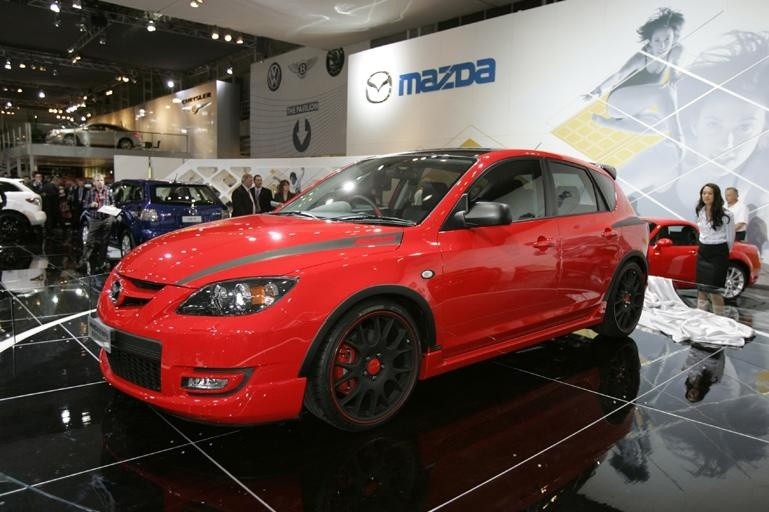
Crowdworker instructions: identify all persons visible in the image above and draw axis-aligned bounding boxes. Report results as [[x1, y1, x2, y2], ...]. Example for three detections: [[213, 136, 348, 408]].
[[615, 25, 769, 218], [250, 174, 273, 214], [273, 180, 296, 205], [610, 414, 652, 486], [289, 172, 301, 194], [724, 187, 748, 242], [682, 342, 726, 402], [24, 170, 116, 272], [695, 183, 736, 317], [231, 174, 257, 216], [579, 4, 685, 163], [629, 328, 769, 478]]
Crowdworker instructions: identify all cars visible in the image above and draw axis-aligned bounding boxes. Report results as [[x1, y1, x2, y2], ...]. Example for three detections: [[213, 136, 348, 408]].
[[43, 121, 145, 150], [636, 218, 761, 302], [80, 179, 229, 264], [93, 148, 650, 432], [0, 178, 46, 246]]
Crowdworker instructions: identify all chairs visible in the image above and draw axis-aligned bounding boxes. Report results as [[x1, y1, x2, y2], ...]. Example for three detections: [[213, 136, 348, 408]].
[[404, 180, 453, 229]]
